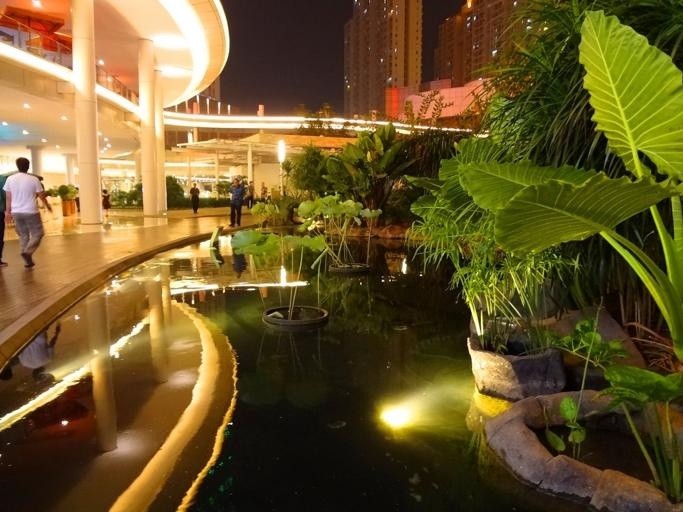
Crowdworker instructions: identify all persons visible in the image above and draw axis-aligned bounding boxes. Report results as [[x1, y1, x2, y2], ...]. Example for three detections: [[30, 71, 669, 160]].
[[101, 189, 111, 217], [0, 174, 8, 267], [244, 181, 256, 210], [1, 157, 53, 267], [189, 183, 199, 214], [227, 175, 244, 227], [259, 181, 267, 203], [74, 187, 79, 212]]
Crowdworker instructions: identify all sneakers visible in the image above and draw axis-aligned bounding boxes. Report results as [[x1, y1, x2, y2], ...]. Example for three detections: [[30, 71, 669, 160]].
[[0, 258, 8, 267], [21, 251, 36, 269], [228, 223, 241, 227]]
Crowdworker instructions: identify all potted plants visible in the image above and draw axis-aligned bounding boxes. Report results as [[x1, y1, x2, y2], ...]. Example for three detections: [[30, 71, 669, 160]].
[[58, 184, 79, 216], [232, 190, 383, 336]]
